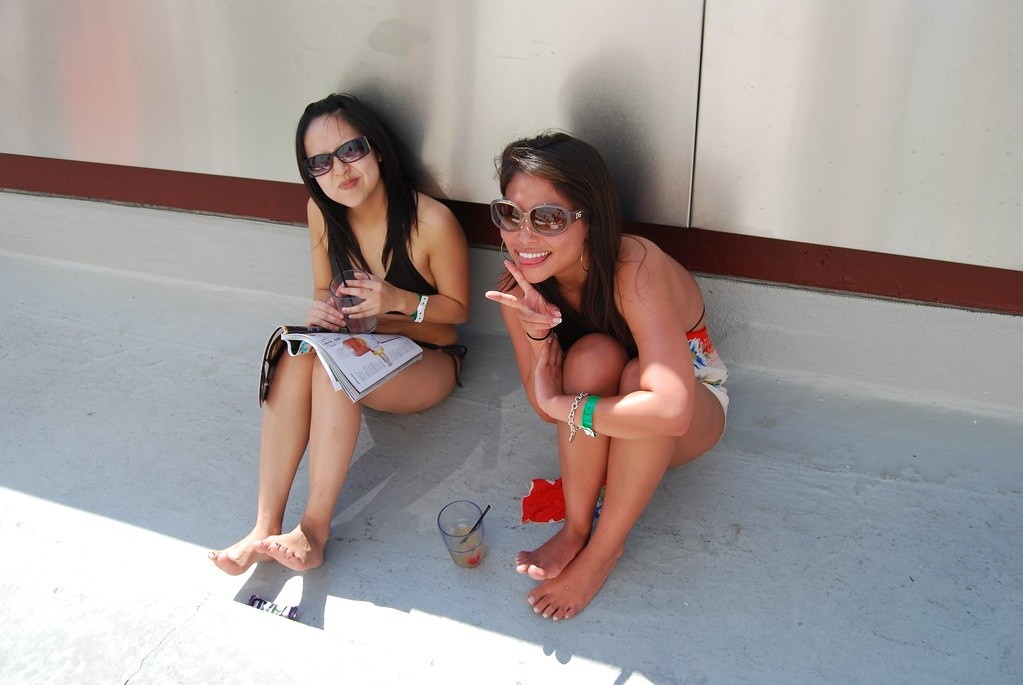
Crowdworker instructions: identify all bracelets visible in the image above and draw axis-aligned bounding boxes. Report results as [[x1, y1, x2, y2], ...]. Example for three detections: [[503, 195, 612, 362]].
[[525, 328, 553, 341], [411, 292, 430, 324], [568, 392, 602, 443]]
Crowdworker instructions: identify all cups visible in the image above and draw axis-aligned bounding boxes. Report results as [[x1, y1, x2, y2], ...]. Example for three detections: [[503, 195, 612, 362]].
[[437, 500, 484, 568], [329, 270, 376, 333]]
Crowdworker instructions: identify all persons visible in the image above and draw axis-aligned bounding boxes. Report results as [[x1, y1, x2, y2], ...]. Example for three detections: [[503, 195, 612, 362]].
[[485, 127, 729, 622], [206, 93, 471, 576]]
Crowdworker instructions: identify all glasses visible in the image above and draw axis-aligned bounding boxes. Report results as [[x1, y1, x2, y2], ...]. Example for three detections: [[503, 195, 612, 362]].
[[489, 198, 590, 236], [303, 135, 374, 179]]
